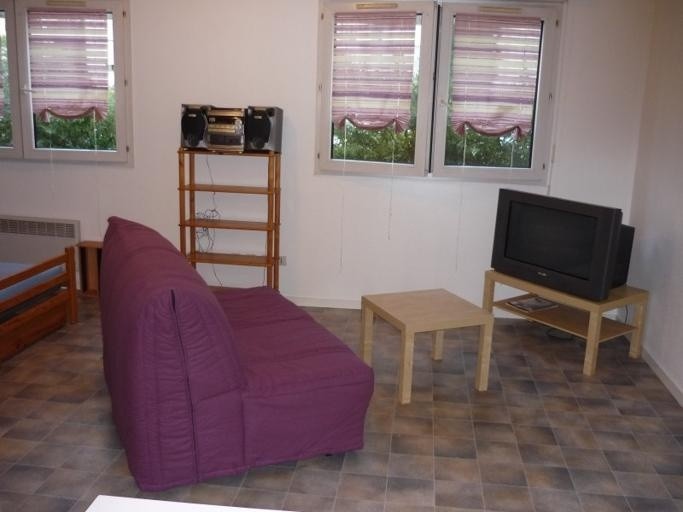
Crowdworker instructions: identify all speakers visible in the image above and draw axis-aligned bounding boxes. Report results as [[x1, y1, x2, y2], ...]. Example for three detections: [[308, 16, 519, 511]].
[[182, 104, 214, 151], [245, 106, 282, 154]]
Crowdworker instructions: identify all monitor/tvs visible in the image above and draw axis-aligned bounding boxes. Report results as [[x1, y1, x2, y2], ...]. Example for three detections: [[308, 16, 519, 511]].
[[490, 189, 635, 300]]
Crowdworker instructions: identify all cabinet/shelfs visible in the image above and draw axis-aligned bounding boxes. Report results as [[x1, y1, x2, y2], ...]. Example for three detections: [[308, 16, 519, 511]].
[[75, 238, 105, 299], [178, 148, 282, 292]]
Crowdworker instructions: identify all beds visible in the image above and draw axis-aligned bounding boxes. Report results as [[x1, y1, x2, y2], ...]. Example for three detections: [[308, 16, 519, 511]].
[[0, 242, 78, 362]]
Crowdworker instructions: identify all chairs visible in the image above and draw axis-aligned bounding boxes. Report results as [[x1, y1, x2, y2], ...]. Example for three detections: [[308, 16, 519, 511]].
[[98, 217, 375, 492]]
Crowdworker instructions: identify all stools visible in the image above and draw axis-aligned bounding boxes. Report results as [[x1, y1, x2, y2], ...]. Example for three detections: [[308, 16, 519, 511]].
[[361, 288, 495, 403]]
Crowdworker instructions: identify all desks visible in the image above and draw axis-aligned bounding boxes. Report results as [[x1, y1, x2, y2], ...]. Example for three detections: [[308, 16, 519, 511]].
[[483, 269, 650, 375]]
